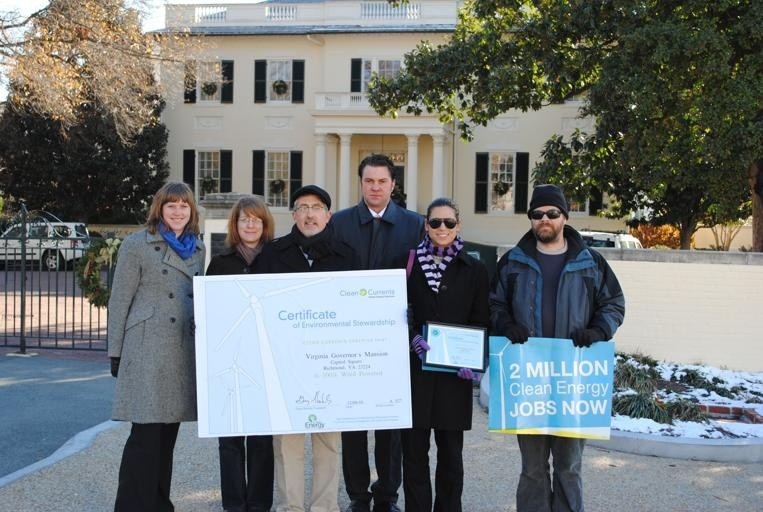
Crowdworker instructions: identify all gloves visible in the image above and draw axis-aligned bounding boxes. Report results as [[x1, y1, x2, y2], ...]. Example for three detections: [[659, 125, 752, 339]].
[[457, 368, 480, 386], [412, 335, 429, 359], [571, 329, 599, 347], [110, 357, 119, 376], [503, 322, 528, 343]]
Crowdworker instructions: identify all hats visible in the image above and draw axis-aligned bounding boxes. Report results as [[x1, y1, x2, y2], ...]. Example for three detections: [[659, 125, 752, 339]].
[[290, 185, 330, 207], [528, 185, 568, 218]]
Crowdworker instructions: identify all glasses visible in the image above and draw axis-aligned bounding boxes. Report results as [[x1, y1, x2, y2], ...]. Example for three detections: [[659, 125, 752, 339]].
[[530, 210, 561, 219], [296, 205, 325, 212], [427, 218, 457, 228], [237, 217, 262, 224]]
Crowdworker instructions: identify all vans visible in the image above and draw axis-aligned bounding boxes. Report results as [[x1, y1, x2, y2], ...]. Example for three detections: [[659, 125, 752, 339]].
[[576, 230, 643, 249], [1, 223, 91, 271]]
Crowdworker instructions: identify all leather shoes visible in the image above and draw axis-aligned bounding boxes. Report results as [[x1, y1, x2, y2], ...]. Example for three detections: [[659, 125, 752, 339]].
[[347, 502, 370, 511], [373, 503, 400, 512]]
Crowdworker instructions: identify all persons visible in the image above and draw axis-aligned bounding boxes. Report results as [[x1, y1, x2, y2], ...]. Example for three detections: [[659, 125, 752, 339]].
[[487, 184, 624, 512], [108, 181, 206, 512], [262, 185, 352, 512], [402, 197, 490, 512], [205, 196, 274, 512], [330, 154, 427, 512]]
